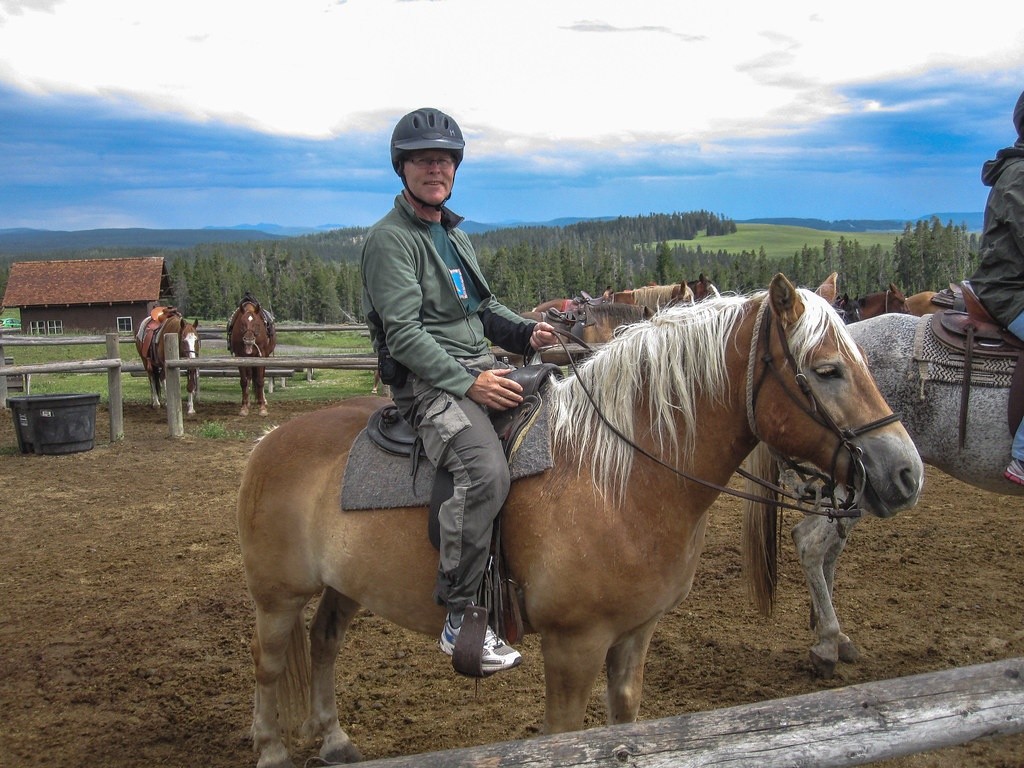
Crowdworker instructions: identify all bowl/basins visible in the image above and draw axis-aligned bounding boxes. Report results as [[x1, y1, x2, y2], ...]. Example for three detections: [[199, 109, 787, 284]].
[[6, 393, 100, 454]]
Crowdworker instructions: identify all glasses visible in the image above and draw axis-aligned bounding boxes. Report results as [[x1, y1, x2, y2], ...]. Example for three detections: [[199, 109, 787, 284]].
[[404, 156, 457, 169]]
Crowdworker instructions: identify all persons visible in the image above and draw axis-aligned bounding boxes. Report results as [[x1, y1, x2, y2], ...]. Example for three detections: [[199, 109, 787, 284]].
[[969, 90, 1024, 484], [359, 109, 556, 672]]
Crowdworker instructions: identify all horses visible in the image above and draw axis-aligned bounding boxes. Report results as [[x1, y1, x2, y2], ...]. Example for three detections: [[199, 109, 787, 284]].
[[238, 270, 1024, 767], [227, 291, 277, 416], [133, 306, 201, 414]]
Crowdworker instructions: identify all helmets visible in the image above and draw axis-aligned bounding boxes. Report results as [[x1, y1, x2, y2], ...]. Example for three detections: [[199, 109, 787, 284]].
[[391, 108, 465, 177]]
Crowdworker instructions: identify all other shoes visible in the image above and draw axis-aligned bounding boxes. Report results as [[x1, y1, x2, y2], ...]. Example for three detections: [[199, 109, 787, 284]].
[[1004, 458, 1024, 485]]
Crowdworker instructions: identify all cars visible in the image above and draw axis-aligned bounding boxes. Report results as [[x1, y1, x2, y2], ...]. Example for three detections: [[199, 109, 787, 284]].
[[0, 318, 21, 328]]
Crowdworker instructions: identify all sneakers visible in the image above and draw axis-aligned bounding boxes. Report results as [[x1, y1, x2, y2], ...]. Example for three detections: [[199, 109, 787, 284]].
[[440, 612, 523, 672]]
